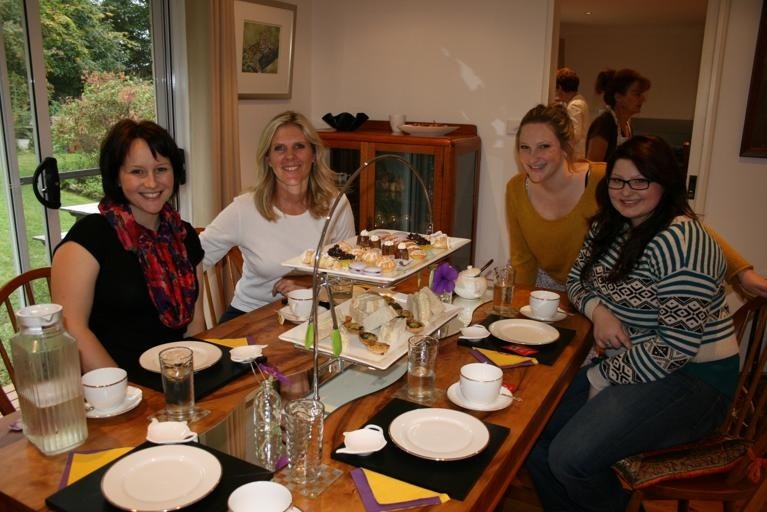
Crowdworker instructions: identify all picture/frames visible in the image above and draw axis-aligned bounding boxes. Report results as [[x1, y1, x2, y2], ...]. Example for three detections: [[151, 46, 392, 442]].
[[739, 0, 767, 157], [237, 0, 297, 100]]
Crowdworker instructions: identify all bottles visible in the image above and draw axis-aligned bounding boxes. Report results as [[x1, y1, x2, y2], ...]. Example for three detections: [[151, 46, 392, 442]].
[[492, 264, 515, 312]]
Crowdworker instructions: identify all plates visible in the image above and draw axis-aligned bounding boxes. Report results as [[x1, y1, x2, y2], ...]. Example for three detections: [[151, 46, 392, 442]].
[[100, 444, 225, 511], [398, 124, 461, 137], [446, 376, 516, 413], [229, 344, 275, 363], [141, 340, 224, 376], [489, 318, 561, 346], [277, 287, 463, 372], [278, 305, 328, 324], [336, 424, 387, 456], [277, 228, 474, 287], [388, 404, 494, 460], [142, 420, 197, 446], [79, 386, 143, 417], [519, 304, 567, 324]]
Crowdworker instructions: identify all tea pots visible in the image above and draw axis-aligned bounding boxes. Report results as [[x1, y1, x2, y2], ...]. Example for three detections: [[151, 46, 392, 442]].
[[9, 303, 90, 456]]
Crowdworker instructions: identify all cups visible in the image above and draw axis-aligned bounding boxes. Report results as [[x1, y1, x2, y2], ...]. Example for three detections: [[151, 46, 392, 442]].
[[282, 399, 326, 484], [333, 173, 353, 186], [159, 347, 199, 415], [388, 114, 406, 133], [82, 366, 128, 409], [406, 335, 440, 403], [377, 209, 414, 229], [458, 362, 504, 405], [228, 481, 292, 512]]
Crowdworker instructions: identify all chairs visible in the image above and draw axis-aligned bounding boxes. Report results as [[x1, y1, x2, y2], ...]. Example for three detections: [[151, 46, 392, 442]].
[[610, 297, 767, 512]]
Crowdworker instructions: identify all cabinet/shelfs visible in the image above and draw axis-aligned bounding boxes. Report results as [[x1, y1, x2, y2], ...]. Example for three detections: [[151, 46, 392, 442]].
[[317, 121, 480, 270]]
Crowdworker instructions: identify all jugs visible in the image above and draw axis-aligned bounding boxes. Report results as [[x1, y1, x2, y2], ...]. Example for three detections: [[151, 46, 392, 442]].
[[452, 265, 488, 301]]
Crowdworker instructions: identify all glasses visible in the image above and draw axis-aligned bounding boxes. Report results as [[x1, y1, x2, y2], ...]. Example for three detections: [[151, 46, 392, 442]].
[[604, 177, 655, 191]]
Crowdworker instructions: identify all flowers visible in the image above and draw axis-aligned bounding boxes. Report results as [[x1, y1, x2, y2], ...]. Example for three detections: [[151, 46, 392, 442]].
[[249, 357, 290, 467], [428, 263, 457, 336]]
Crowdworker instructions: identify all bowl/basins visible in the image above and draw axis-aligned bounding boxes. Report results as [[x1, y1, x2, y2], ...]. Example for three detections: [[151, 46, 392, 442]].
[[529, 290, 561, 319], [287, 289, 318, 319]]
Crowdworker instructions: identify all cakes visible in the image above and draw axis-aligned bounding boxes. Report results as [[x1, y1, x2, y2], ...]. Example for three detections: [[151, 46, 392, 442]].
[[302, 228, 448, 277]]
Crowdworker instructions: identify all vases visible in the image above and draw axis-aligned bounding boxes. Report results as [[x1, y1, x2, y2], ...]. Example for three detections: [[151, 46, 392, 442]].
[[430, 286, 453, 339]]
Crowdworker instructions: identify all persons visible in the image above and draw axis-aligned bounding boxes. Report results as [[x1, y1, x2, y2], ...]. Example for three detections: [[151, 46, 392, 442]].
[[193, 111, 358, 330], [51, 118, 207, 384], [519, 132, 739, 511], [584, 68, 650, 164], [504, 102, 765, 303], [555, 68, 593, 161]]
